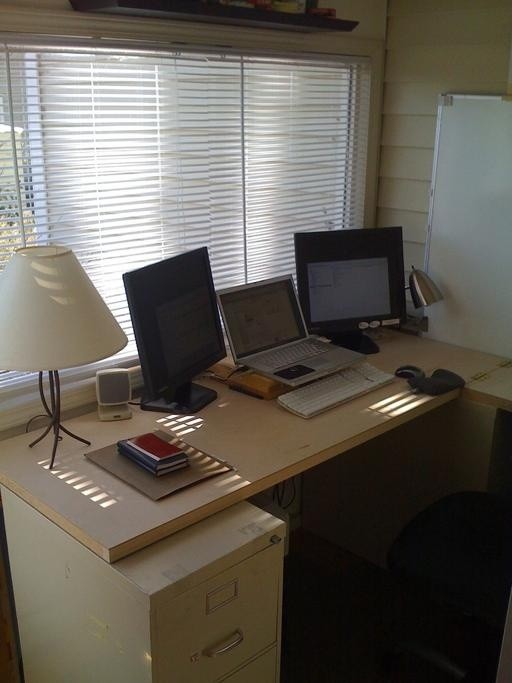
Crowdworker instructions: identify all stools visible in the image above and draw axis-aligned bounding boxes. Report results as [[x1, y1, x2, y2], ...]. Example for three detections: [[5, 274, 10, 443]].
[[378, 489, 512, 682]]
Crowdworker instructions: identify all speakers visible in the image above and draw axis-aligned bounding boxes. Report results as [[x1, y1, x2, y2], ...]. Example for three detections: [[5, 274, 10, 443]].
[[97, 368, 132, 420]]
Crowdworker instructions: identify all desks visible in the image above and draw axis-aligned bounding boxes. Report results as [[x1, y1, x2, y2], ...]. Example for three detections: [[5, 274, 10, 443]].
[[1, 323, 510, 568]]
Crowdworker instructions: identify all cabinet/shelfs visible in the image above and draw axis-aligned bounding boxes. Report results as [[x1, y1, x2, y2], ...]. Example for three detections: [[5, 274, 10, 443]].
[[1, 480, 291, 682]]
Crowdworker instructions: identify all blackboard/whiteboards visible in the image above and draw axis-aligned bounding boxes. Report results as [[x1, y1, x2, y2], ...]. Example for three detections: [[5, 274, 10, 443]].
[[419, 91, 512, 360]]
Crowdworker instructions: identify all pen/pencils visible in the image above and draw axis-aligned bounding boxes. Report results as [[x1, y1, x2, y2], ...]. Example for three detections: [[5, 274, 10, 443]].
[[229, 384, 264, 399]]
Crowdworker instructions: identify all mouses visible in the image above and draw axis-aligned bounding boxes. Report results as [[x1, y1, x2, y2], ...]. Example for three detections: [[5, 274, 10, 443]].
[[396, 365, 426, 379]]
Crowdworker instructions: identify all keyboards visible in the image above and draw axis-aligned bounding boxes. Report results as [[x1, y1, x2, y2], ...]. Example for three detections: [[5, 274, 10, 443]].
[[276, 362, 395, 418]]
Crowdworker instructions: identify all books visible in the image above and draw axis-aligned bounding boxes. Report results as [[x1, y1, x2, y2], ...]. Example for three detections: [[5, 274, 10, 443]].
[[126, 432, 184, 462], [116, 447, 190, 477], [118, 436, 188, 470]]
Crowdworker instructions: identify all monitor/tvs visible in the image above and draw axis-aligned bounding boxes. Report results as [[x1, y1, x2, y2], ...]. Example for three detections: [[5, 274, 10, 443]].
[[295, 226, 406, 354], [122, 246, 228, 414]]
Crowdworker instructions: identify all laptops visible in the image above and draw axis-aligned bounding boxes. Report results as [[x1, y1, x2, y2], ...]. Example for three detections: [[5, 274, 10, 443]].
[[217, 274, 366, 387]]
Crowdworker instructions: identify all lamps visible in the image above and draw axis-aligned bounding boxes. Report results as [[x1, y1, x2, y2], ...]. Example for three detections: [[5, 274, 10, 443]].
[[400, 263, 454, 321], [2, 243, 134, 472]]
